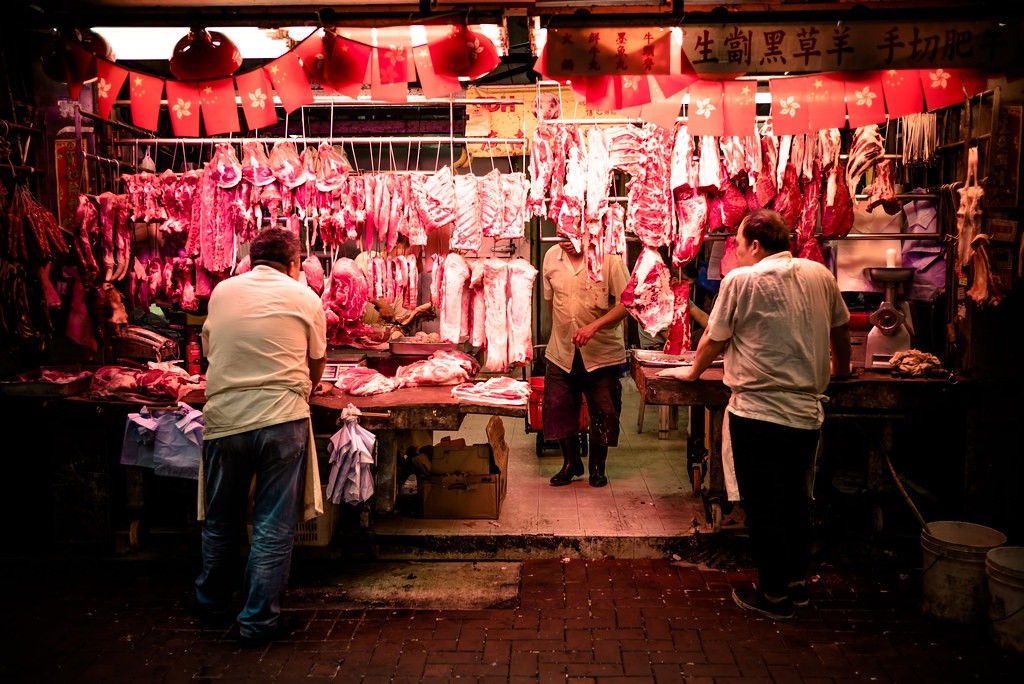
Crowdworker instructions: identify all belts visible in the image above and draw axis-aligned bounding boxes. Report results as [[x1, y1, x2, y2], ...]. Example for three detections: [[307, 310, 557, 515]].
[[644, 343, 665, 350]]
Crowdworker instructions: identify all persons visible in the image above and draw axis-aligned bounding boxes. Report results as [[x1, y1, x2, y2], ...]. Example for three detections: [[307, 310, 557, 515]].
[[539, 219, 631, 487], [650, 207, 854, 620], [637, 264, 710, 350], [187, 229, 332, 641]]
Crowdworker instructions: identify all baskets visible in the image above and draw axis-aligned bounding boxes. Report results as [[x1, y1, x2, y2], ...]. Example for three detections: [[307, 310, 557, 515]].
[[528, 377, 589, 431], [246, 433, 340, 547]]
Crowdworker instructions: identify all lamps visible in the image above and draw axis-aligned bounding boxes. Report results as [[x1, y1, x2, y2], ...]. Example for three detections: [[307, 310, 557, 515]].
[[42, 28, 107, 82], [430, 5, 500, 81], [169, 9, 244, 94], [303, 7, 356, 87]]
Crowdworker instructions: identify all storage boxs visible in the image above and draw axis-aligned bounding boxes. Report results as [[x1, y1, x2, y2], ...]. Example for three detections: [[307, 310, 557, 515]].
[[246, 498, 341, 546], [425, 415, 510, 519], [388, 337, 453, 354]]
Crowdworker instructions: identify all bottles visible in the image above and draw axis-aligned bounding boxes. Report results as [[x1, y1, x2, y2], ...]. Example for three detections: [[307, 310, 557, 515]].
[[187, 334, 202, 377]]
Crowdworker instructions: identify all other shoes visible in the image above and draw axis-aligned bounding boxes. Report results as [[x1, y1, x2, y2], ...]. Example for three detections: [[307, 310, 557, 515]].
[[194, 584, 244, 616], [236, 611, 299, 648], [751, 577, 810, 606], [731, 588, 795, 621]]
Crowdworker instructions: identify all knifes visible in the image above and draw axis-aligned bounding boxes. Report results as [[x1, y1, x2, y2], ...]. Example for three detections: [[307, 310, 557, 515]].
[[374, 304, 414, 334]]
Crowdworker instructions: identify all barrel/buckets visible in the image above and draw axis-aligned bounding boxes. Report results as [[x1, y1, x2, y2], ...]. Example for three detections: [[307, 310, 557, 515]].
[[919, 520, 1006, 625], [984, 545, 1024, 655]]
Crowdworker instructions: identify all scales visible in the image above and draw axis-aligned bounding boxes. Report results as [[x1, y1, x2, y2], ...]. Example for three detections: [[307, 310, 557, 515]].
[[322, 353, 368, 382]]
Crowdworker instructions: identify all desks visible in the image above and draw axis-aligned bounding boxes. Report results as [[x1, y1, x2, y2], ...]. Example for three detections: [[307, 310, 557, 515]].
[[630, 356, 981, 533]]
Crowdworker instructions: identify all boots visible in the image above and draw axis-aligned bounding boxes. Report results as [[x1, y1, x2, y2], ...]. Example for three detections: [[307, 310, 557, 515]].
[[588, 435, 607, 487], [550, 433, 584, 484]]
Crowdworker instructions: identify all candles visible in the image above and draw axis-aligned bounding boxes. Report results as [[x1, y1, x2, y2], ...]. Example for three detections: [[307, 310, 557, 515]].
[[887, 249, 896, 268]]
